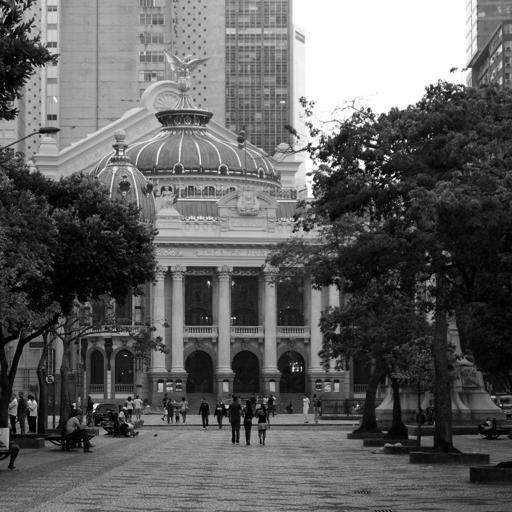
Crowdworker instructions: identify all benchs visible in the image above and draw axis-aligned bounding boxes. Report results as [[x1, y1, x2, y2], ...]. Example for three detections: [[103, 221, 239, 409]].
[[43, 420, 96, 449]]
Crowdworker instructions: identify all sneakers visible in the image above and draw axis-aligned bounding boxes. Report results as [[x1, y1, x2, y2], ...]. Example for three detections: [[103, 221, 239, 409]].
[[232, 442, 235, 444], [84, 449, 90, 452], [259, 441, 265, 445], [236, 443, 239, 446], [247, 440, 250, 445], [130, 431, 138, 438], [202, 427, 206, 429], [88, 443, 94, 447]]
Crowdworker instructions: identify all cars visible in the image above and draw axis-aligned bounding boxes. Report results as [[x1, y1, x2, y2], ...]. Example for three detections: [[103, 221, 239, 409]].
[[489, 394, 512, 416]]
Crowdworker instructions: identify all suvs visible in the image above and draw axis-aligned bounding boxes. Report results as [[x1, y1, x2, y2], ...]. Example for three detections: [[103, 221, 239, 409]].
[[94, 402, 119, 426], [222, 398, 268, 417]]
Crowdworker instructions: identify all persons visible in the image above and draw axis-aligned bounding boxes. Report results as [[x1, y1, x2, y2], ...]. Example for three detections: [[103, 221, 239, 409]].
[[161, 393, 189, 424], [312, 394, 323, 424], [416, 409, 427, 424], [8, 393, 38, 432], [286, 400, 294, 413], [65, 411, 97, 452], [199, 394, 277, 446], [117, 394, 144, 438], [302, 393, 311, 424], [87, 396, 95, 426]]
